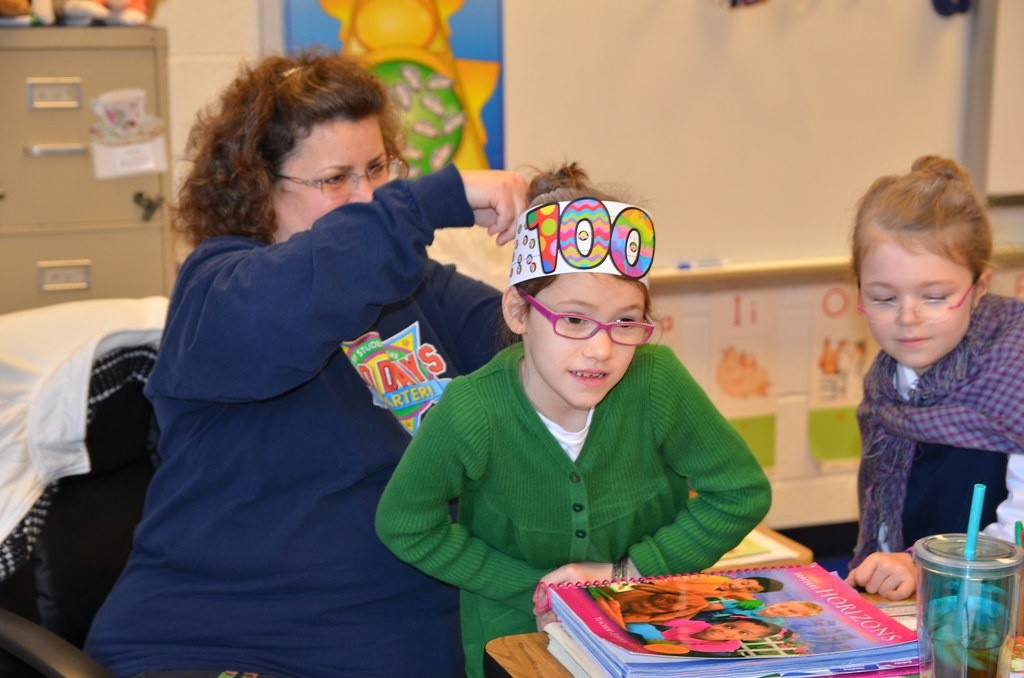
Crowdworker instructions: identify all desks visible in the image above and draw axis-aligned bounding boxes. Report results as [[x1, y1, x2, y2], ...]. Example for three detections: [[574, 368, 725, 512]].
[[699, 524, 813, 574], [482, 592, 1023, 678]]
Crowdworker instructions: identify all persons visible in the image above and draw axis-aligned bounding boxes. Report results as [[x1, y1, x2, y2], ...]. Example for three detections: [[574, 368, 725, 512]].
[[650, 611, 792, 654], [823, 152, 1024, 605], [690, 597, 824, 627], [78, 44, 534, 677], [374, 164, 770, 678], [636, 574, 785, 604]]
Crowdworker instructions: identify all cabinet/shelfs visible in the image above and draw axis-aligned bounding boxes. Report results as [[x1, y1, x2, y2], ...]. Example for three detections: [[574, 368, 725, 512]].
[[0, 26, 176, 314]]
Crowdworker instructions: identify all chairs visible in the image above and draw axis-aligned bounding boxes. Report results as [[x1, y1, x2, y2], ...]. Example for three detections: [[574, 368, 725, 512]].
[[0, 328, 162, 678]]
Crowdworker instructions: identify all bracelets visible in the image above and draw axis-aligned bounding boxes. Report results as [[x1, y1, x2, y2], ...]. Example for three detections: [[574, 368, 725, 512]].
[[611, 556, 628, 584]]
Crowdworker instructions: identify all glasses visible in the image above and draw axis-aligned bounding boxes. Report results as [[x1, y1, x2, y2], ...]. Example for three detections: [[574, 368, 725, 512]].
[[273, 152, 411, 198], [858, 273, 978, 326], [520, 291, 656, 346]]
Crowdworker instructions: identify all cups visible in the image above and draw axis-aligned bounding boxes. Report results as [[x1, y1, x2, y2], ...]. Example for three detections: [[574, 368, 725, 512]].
[[912, 532, 1024, 678]]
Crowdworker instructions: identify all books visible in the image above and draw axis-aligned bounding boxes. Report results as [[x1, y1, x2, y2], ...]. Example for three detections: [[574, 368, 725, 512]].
[[543, 561, 936, 678]]
